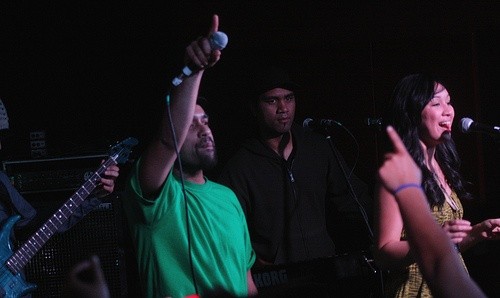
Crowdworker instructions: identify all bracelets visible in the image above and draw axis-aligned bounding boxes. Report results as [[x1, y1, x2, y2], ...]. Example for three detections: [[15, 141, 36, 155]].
[[393, 183, 424, 194]]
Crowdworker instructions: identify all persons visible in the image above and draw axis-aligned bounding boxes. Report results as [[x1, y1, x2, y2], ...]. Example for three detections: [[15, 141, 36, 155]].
[[120, 13, 260, 298], [0, 98, 120, 298], [372, 73, 500, 298], [215, 79, 374, 267]]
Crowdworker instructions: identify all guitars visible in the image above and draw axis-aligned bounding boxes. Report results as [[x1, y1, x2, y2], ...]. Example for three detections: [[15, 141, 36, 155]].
[[0, 136, 141, 298]]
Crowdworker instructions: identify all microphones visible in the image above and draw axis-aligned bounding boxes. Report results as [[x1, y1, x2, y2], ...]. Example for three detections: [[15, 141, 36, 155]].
[[303, 118, 342, 128], [458, 118, 500, 134], [171, 32, 228, 90]]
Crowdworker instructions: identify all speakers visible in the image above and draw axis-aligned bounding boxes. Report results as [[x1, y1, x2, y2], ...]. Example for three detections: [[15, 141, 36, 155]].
[[10, 200, 141, 298]]
